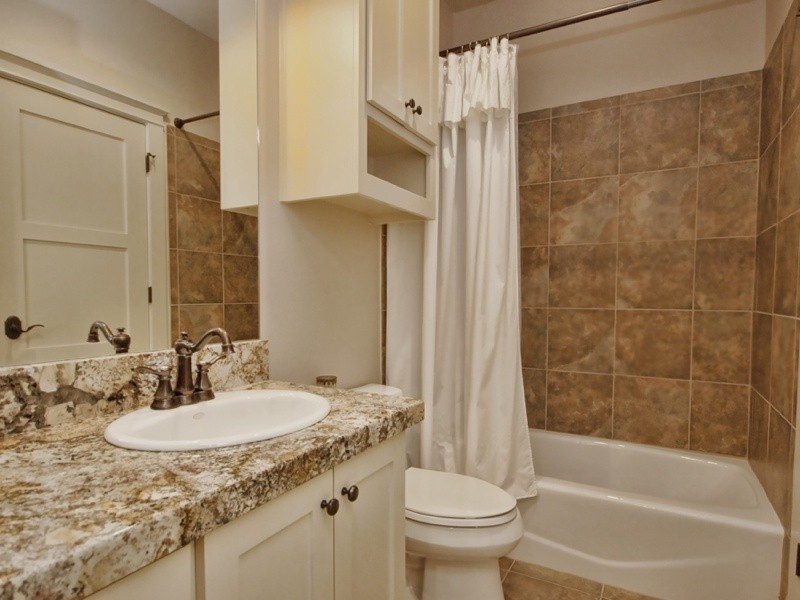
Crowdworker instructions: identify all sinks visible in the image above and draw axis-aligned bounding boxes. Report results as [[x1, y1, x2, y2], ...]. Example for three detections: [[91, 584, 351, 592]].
[[103, 390, 332, 452]]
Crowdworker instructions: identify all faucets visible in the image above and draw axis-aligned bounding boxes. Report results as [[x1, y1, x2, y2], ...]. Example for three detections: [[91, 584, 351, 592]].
[[85, 321, 131, 354], [174, 327, 236, 406]]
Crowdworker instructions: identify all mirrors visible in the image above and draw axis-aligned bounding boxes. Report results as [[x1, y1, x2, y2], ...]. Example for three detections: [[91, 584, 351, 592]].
[[0, 0, 262, 368]]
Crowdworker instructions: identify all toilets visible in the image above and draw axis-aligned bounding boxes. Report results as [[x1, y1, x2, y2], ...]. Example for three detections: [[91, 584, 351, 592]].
[[348, 382, 524, 600]]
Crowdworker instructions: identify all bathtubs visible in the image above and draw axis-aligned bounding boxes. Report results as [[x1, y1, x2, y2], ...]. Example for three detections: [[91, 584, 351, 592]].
[[504, 426, 786, 600]]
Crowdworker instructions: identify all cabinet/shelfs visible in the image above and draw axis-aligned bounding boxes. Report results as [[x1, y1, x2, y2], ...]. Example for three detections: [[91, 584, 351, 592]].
[[197, 427, 411, 600], [216, 0, 262, 217], [277, 0, 441, 219]]
